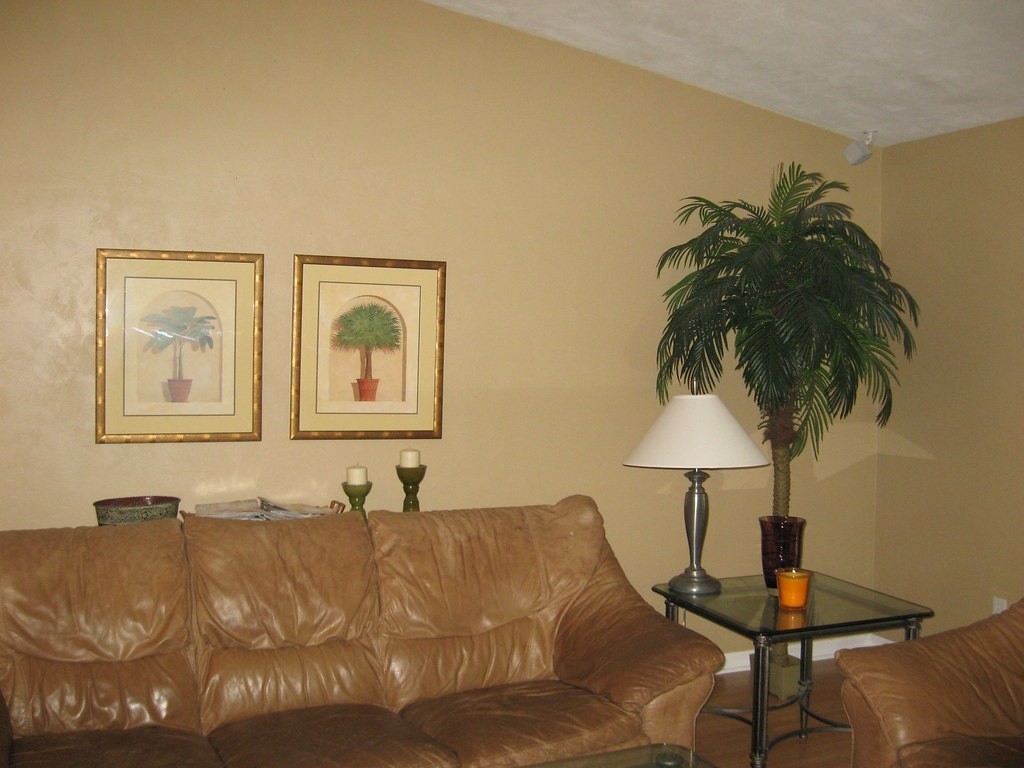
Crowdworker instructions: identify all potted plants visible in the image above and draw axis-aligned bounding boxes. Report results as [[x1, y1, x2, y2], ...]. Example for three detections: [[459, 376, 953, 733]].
[[652, 160, 921, 703]]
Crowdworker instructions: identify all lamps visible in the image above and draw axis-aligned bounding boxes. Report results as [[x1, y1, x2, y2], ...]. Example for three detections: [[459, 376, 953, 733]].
[[622, 394, 772, 596]]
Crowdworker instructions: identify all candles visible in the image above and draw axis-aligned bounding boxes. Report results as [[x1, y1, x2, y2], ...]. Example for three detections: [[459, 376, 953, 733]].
[[346, 460, 369, 486], [400, 444, 420, 469]]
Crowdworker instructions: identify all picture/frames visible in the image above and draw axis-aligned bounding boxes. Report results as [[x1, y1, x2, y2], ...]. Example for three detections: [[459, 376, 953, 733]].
[[94, 246, 267, 445], [288, 253, 448, 441]]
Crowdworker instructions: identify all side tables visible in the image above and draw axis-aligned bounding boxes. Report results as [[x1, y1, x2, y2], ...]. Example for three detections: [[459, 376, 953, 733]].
[[650, 567, 935, 768]]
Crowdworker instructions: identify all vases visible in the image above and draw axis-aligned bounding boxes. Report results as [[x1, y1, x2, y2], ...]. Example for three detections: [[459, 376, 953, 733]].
[[92, 496, 182, 527]]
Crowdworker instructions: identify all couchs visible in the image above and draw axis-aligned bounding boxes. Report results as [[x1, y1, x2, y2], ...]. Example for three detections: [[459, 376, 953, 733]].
[[0, 493, 727, 768], [834, 597, 1023, 768]]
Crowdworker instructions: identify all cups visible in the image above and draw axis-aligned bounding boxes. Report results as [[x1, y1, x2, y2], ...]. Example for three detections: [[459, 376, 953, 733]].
[[774, 568, 814, 611]]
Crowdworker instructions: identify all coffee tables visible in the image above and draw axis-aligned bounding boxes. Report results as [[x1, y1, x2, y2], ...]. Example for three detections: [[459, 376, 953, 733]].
[[518, 743, 717, 768]]
